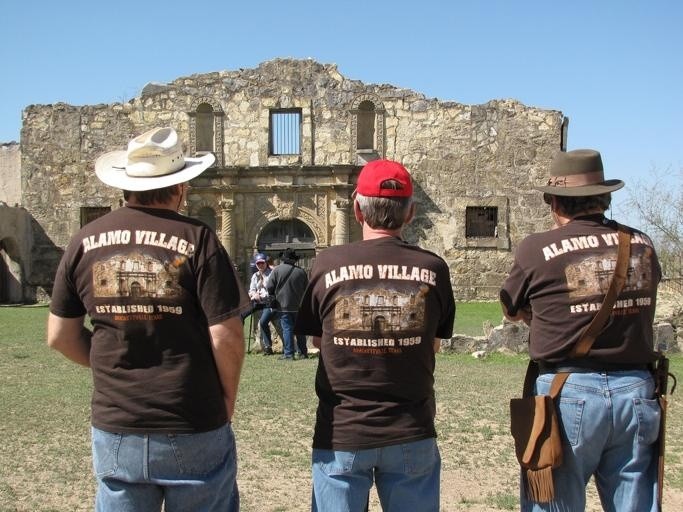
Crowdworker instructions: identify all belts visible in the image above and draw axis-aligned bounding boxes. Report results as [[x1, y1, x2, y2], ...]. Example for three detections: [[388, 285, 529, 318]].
[[541, 364, 647, 373]]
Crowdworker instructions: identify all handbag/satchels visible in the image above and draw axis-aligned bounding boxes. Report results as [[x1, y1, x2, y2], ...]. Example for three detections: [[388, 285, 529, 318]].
[[509, 396, 564, 469]]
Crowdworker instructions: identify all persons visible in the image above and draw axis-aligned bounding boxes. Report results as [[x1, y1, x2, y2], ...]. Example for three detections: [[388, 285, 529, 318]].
[[235, 246, 310, 360], [292, 160, 454, 512], [498, 149, 663, 512], [44, 125, 245, 512]]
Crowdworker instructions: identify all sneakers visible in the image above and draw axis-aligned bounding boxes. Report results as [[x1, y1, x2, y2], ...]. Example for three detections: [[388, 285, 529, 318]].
[[263, 347, 309, 360]]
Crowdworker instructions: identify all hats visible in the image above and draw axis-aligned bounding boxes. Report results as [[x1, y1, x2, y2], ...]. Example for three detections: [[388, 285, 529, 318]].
[[94, 126, 216, 192], [535, 149, 626, 198], [351, 160, 414, 198], [255, 253, 266, 263], [279, 248, 301, 264]]
